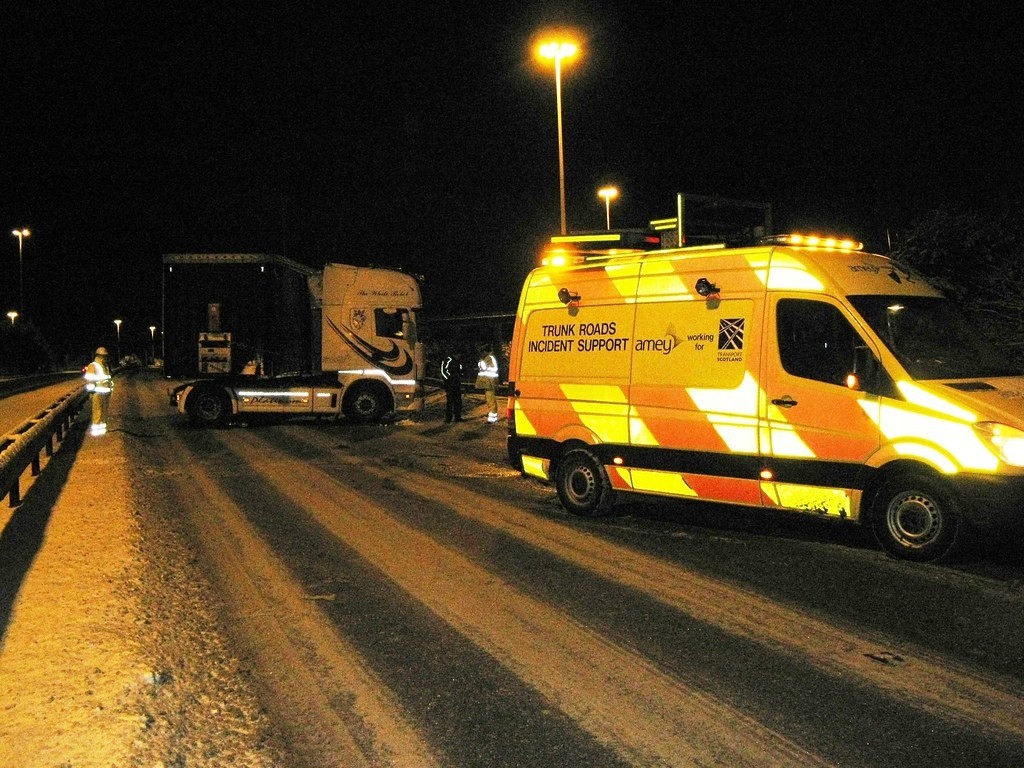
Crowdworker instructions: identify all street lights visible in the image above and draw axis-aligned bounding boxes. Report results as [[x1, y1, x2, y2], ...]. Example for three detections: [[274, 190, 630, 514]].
[[598, 187, 618, 230], [534, 29, 579, 236], [114, 319, 123, 365], [148, 325, 157, 360], [13, 228, 30, 313]]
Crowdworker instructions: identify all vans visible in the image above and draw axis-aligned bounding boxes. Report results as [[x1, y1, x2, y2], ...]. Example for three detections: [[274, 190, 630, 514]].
[[507, 236, 1024, 566]]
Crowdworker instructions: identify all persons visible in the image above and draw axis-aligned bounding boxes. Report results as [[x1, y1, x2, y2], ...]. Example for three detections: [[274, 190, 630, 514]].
[[441, 348, 468, 423], [478, 344, 498, 422], [85, 347, 114, 436]]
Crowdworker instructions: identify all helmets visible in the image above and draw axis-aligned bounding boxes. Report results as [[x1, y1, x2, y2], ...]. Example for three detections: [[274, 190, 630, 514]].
[[97, 347, 108, 355]]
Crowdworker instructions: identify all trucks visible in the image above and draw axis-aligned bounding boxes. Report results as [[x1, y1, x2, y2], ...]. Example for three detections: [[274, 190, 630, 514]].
[[168, 263, 430, 428]]
